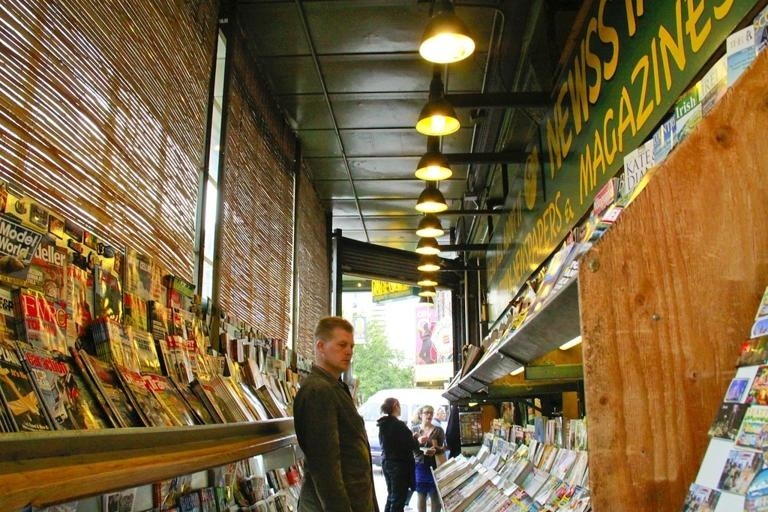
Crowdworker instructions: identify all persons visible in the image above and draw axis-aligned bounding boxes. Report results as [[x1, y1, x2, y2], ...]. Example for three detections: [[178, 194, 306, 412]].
[[376, 397, 421, 511], [294, 317, 379, 512], [411, 403, 449, 512]]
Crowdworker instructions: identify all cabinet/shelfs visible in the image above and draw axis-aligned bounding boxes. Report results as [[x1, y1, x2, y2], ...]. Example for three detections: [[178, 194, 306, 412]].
[[428, 44, 767, 512]]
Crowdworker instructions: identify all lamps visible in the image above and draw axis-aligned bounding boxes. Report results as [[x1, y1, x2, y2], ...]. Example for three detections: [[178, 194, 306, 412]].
[[416, 0, 478, 306]]
